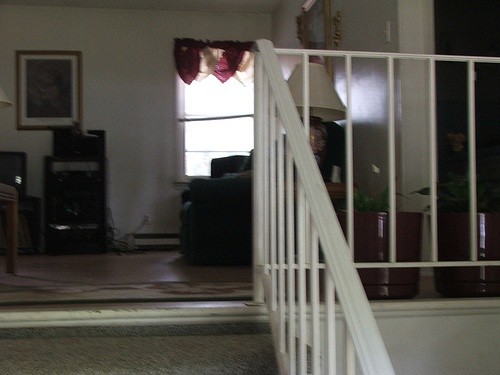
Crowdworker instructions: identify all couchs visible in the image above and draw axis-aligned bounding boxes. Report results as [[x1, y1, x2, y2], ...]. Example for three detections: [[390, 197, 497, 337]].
[[179, 124, 351, 267]]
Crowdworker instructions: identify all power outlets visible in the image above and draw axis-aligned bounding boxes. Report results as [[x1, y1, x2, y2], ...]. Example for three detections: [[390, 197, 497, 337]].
[[142, 214, 151, 226]]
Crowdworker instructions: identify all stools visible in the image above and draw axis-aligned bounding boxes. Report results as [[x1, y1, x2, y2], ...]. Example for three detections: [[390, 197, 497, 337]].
[[0, 183, 18, 277]]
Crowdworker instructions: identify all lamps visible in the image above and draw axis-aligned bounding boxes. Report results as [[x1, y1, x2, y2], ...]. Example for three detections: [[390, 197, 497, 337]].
[[275, 62, 347, 123]]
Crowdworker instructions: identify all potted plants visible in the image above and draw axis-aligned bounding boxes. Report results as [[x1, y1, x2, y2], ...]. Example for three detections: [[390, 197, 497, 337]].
[[334, 175, 425, 301], [428, 176, 500, 299]]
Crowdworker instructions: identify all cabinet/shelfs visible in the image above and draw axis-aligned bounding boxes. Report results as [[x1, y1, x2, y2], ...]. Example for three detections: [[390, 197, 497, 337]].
[[44, 156, 107, 256]]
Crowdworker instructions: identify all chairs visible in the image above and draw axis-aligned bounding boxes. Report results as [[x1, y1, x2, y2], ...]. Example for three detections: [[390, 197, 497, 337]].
[[0, 150, 43, 256]]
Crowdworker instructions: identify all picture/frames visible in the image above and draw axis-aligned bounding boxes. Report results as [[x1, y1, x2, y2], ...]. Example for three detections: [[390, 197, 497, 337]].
[[15, 49, 85, 131], [295, 0, 333, 84]]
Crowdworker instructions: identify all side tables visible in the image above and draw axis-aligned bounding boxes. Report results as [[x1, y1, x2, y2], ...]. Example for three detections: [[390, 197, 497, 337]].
[[282, 182, 348, 196]]
